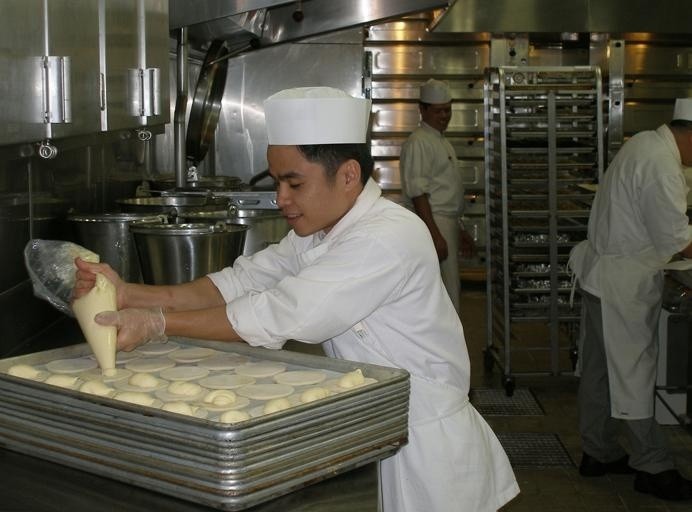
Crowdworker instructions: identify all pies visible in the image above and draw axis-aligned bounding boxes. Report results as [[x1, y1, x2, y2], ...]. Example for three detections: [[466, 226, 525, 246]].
[[8, 340, 379, 426]]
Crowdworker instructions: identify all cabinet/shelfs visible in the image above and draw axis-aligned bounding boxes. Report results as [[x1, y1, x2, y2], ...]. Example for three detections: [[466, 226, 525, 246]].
[[484, 66, 603, 398], [0, 0, 170, 160]]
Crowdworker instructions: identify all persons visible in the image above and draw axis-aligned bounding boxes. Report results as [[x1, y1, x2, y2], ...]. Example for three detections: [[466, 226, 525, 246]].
[[399, 78, 475, 403], [569, 94, 691, 501], [66, 86, 521, 512]]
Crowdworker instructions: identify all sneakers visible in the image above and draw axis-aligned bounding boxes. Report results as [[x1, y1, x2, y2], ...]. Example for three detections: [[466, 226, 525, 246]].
[[634, 468, 692, 502], [579, 452, 637, 477]]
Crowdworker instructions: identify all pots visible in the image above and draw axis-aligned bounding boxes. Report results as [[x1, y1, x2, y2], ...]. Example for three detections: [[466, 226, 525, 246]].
[[65, 175, 289, 285]]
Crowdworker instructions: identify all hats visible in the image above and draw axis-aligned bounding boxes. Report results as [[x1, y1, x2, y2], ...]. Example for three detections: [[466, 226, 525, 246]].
[[419, 79, 452, 104], [673, 97, 692, 121], [263, 87, 372, 145]]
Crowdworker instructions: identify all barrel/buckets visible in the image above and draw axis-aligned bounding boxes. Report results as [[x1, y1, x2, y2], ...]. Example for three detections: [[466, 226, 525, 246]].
[[133, 221, 249, 288]]
